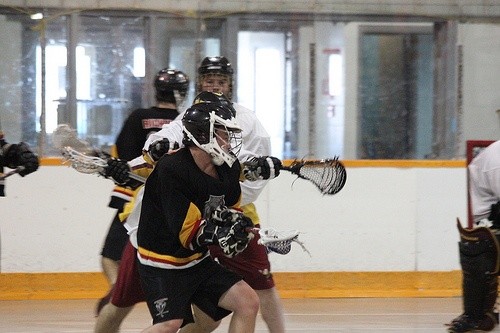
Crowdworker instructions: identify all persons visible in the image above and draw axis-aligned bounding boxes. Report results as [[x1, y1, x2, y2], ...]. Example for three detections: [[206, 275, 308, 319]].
[[93, 54, 290, 333], [0, 127, 39, 196], [445, 140, 500, 333]]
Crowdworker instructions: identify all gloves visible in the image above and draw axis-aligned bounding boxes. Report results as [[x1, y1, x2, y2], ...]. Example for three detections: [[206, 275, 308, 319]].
[[104, 157, 130, 185], [245, 155, 280, 181], [211, 206, 254, 258], [6, 141, 39, 176], [148, 138, 179, 161]]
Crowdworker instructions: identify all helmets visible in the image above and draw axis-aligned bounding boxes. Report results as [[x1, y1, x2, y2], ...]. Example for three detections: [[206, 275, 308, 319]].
[[192, 91, 237, 120], [197, 56, 233, 96], [182, 103, 243, 164], [154, 67, 190, 92]]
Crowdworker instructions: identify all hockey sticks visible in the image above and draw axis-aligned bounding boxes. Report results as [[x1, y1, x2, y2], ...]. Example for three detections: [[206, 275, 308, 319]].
[[51, 123, 114, 159], [62, 146, 148, 185], [0, 166, 24, 183], [242, 156, 348, 196], [244, 227, 301, 256]]
